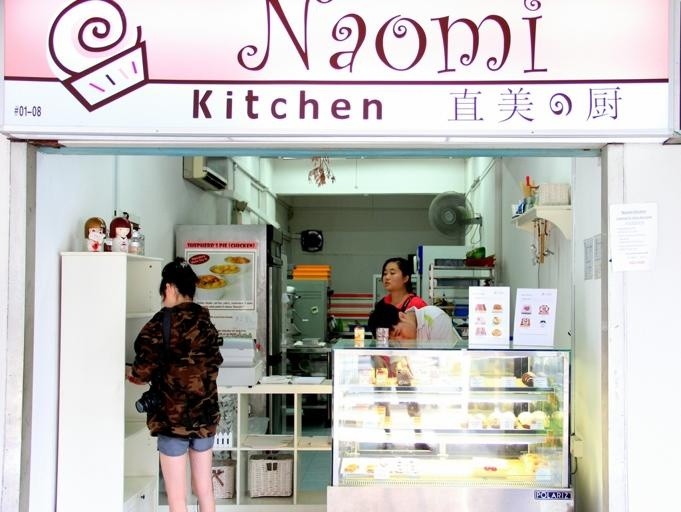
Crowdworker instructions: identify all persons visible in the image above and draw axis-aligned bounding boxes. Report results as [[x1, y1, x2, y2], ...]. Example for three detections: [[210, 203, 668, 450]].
[[85, 216, 131, 252], [368, 258, 463, 341], [126, 256, 223, 512]]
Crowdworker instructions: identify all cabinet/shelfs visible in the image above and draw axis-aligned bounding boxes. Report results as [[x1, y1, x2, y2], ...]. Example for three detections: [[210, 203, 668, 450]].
[[54, 250, 164, 512], [159, 380, 331, 511], [427, 263, 495, 339], [325, 343, 576, 512]]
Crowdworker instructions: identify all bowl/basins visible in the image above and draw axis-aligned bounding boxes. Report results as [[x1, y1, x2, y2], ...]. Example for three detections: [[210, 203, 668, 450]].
[[348, 323, 369, 331], [246, 417, 270, 434]]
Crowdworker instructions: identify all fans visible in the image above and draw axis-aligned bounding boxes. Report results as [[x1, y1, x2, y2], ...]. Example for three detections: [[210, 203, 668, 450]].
[[428, 191, 483, 243]]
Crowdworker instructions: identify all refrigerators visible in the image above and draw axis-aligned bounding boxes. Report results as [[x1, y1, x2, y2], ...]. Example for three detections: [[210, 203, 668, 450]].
[[418, 244, 480, 313], [174, 222, 281, 455]]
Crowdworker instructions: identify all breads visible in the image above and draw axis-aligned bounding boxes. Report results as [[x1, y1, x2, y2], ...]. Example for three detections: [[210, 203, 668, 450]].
[[345, 367, 550, 477]]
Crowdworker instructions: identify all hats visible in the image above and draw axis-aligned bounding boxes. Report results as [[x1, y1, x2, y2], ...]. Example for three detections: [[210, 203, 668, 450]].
[[162, 257, 199, 286]]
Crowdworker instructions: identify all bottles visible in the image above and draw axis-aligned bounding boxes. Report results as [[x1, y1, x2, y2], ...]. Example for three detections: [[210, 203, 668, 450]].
[[338, 318, 343, 331], [102, 228, 112, 253], [131, 232, 144, 256]]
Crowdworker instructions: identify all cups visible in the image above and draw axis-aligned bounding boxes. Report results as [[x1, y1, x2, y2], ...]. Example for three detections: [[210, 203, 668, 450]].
[[376, 327, 389, 342], [354, 328, 365, 341]]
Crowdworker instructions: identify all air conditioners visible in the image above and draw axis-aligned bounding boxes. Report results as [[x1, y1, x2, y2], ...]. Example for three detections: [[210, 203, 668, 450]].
[[183, 157, 230, 193]]
